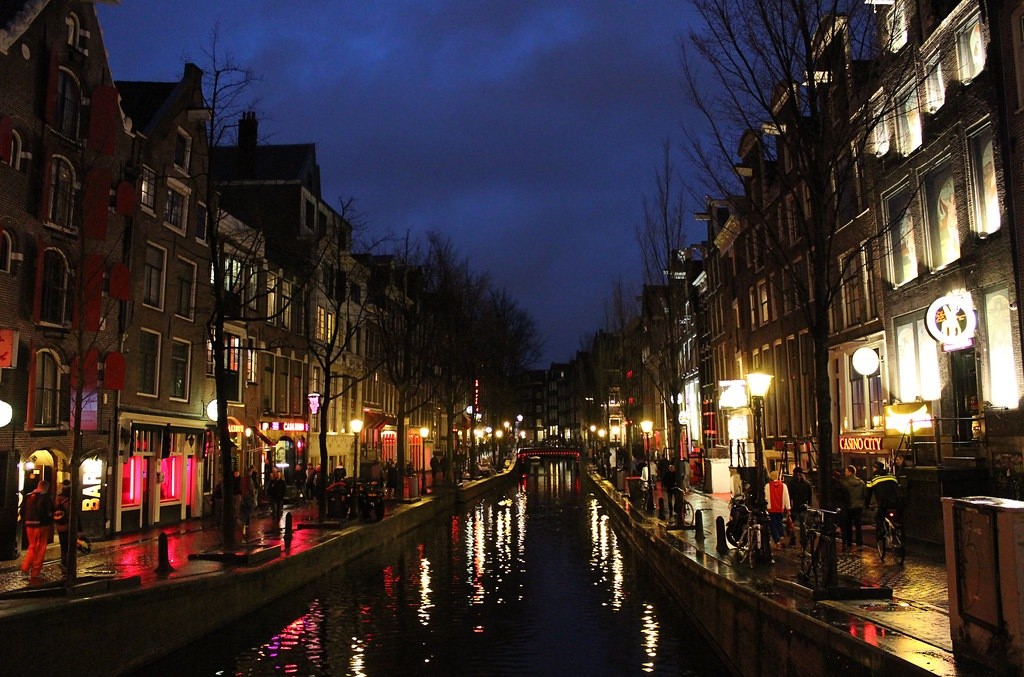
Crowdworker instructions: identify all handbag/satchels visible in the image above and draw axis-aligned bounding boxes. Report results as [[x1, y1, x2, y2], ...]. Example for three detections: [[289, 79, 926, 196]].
[[786, 515, 796, 536]]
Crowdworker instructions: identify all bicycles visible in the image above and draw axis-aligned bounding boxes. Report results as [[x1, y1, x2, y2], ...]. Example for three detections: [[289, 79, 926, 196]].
[[671, 486, 694, 524], [867, 503, 907, 566], [797, 502, 842, 587], [733, 505, 766, 569]]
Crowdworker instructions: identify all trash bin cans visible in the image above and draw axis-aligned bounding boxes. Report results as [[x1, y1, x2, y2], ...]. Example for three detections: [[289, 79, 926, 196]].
[[326, 482, 346, 518], [616, 469, 626, 492], [626, 476, 642, 509], [408, 473, 418, 499]]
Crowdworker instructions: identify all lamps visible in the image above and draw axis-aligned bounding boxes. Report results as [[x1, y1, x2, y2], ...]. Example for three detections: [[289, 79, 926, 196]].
[[930, 267, 937, 274], [893, 284, 899, 290], [892, 399, 900, 405], [121, 427, 131, 446], [915, 396, 925, 403], [978, 232, 988, 239], [983, 401, 1001, 420], [186, 433, 194, 448]]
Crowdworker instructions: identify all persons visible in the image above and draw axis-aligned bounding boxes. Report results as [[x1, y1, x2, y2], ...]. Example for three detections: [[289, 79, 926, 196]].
[[19, 473, 71, 577], [477, 441, 490, 456], [430, 448, 465, 479], [618, 442, 690, 517], [381, 458, 415, 496], [763, 454, 912, 550], [209, 459, 285, 530], [291, 463, 344, 503]]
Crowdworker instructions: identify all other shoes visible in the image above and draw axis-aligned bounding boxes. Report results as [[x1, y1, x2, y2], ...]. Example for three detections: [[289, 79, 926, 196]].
[[789, 537, 796, 545], [33, 573, 46, 578], [776, 542, 782, 550], [858, 545, 864, 550], [780, 536, 785, 545]]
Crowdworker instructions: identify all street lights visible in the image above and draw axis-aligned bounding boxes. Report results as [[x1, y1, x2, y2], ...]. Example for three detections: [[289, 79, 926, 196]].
[[418, 426, 430, 496], [639, 418, 657, 510], [611, 424, 621, 469], [349, 416, 365, 521]]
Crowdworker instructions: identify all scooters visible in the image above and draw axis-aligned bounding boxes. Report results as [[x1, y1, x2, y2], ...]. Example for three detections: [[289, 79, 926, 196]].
[[726, 491, 767, 549]]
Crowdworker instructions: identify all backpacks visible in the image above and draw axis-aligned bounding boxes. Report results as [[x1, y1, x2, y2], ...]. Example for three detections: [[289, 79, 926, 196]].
[[53, 499, 68, 524]]
